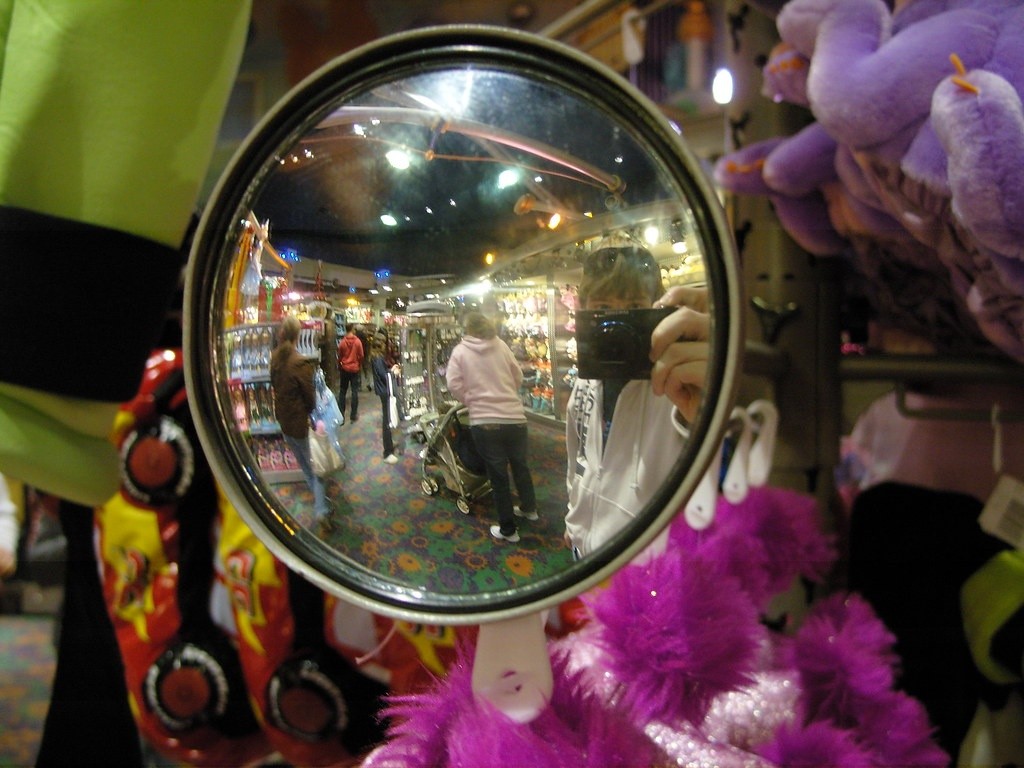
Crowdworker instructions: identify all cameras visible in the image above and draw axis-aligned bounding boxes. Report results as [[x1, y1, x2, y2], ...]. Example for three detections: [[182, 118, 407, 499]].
[[575, 306, 683, 380]]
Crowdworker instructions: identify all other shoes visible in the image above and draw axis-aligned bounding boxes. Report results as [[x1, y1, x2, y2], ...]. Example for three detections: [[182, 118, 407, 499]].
[[367, 385, 371, 391], [351, 415, 359, 421], [384, 453, 399, 464], [315, 515, 333, 532]]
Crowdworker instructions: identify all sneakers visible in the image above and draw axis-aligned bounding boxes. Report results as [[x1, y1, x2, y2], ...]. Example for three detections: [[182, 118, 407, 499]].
[[490, 525, 520, 542], [513, 505, 538, 520]]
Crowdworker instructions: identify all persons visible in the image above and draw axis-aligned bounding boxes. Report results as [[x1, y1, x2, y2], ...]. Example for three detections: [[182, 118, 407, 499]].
[[564, 228, 714, 564], [371, 330, 400, 467], [447, 310, 540, 542], [337, 321, 364, 423], [269, 312, 335, 532]]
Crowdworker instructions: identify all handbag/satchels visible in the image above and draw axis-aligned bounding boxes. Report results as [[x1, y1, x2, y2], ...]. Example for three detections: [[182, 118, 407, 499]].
[[307, 414, 344, 480], [387, 372, 400, 429]]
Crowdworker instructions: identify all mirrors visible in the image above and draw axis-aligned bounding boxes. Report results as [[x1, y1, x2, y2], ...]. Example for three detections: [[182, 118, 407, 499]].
[[182, 27, 742, 625]]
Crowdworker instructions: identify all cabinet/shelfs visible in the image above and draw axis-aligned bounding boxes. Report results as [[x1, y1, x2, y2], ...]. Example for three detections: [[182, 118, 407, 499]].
[[222, 200, 713, 482]]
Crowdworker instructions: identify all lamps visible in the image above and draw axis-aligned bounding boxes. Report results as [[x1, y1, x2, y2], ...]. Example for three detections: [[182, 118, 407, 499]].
[[540, 211, 562, 229], [669, 234, 688, 253], [493, 162, 519, 189]]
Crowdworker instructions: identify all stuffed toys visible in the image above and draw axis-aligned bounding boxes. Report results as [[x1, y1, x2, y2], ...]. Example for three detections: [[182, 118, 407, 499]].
[[714, 0, 1024, 365]]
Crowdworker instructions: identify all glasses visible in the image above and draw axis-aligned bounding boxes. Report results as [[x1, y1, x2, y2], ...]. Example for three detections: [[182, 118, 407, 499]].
[[583, 247, 660, 275]]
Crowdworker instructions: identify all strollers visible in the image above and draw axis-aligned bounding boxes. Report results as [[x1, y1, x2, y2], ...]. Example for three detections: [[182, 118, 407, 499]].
[[418, 402, 494, 517]]
